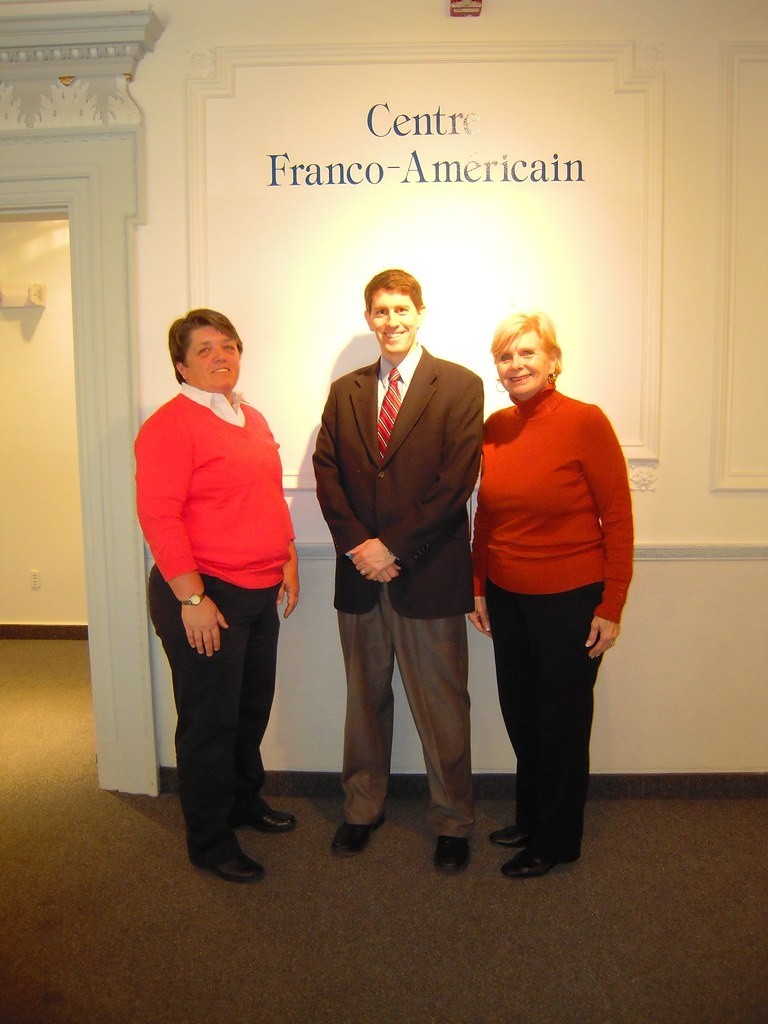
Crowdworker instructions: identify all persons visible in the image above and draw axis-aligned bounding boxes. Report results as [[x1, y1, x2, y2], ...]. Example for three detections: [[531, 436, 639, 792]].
[[135, 309, 300, 883], [312, 269, 484, 874], [468, 313, 634, 878]]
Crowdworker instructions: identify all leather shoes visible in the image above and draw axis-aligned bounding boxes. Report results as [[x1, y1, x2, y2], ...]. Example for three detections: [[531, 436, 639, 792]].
[[247, 808, 295, 832], [332, 821, 369, 856], [434, 836, 471, 873], [501, 848, 557, 878], [190, 851, 264, 882], [490, 825, 531, 847]]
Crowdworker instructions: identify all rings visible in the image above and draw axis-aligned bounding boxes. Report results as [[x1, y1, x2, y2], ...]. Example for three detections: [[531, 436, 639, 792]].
[[610, 642, 615, 647], [362, 570, 366, 574]]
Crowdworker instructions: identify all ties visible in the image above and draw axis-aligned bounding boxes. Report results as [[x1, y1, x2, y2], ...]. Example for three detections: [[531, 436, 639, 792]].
[[378, 368, 401, 461]]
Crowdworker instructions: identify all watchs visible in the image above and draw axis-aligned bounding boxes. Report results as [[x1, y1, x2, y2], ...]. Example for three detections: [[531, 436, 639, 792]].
[[181, 592, 205, 606]]
